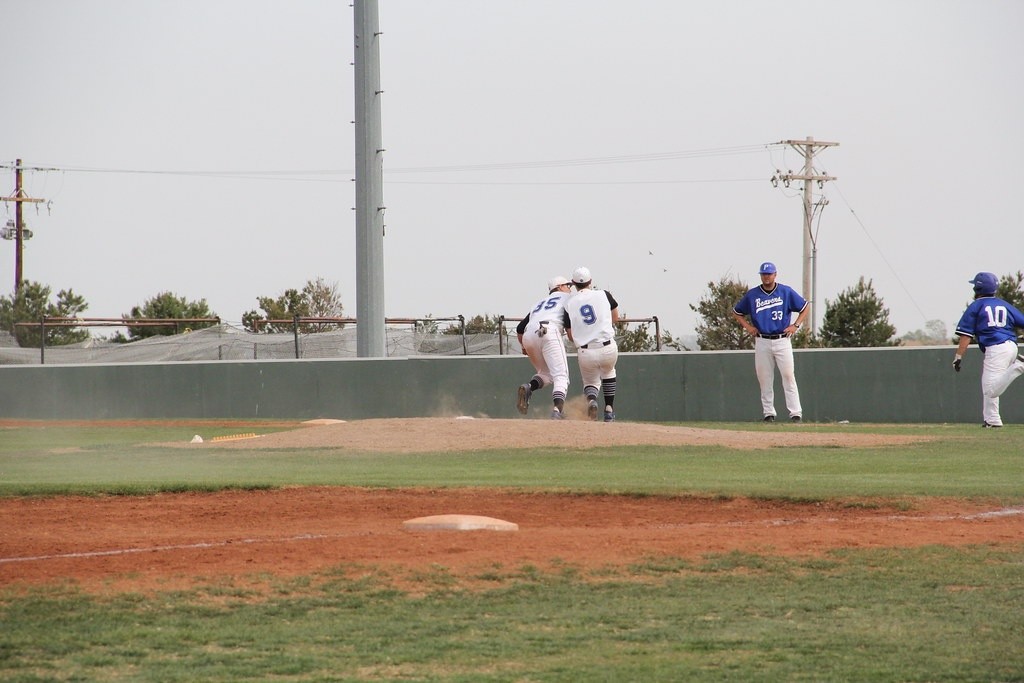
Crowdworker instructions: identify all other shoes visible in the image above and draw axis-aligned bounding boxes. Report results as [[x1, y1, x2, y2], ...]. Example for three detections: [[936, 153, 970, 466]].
[[982, 422, 1000, 428], [792, 416, 801, 423], [1017, 354, 1024, 362], [765, 416, 773, 424]]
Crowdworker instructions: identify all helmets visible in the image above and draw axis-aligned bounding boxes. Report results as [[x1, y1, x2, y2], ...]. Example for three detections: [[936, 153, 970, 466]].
[[757, 262, 777, 274], [968, 272, 998, 295]]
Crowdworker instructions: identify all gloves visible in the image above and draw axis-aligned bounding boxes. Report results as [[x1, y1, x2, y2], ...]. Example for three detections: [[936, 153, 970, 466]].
[[952, 354, 962, 372], [534, 325, 547, 337]]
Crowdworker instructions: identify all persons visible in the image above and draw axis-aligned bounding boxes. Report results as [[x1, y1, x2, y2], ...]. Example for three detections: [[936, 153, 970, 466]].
[[732, 263, 810, 423], [951, 272, 1024, 427], [516, 277, 575, 419], [564, 267, 619, 423]]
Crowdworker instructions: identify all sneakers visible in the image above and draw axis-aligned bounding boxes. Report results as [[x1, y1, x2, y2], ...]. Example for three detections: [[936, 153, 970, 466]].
[[517, 383, 531, 415], [551, 406, 565, 420], [604, 410, 616, 422], [587, 400, 598, 421]]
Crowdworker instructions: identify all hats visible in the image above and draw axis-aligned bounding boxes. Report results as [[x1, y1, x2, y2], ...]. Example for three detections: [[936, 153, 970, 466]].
[[572, 266, 591, 283], [547, 276, 573, 295]]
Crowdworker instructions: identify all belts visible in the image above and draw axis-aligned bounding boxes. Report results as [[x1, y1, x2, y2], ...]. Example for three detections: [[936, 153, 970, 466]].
[[539, 321, 549, 324], [757, 334, 786, 340], [581, 340, 610, 349]]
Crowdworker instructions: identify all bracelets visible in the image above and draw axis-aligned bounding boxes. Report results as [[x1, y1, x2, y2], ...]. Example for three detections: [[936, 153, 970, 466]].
[[794, 323, 800, 328]]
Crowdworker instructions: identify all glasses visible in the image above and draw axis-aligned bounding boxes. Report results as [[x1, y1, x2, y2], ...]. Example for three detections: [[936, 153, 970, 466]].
[[562, 284, 571, 289]]
